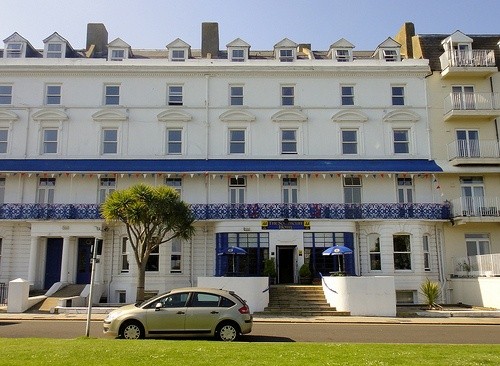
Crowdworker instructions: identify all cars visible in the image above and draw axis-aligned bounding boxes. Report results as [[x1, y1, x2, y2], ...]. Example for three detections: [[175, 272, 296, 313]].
[[104, 287, 253, 341]]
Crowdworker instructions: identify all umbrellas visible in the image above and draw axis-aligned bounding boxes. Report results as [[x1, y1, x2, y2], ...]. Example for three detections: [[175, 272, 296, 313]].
[[217, 245, 247, 256], [322, 244, 354, 256]]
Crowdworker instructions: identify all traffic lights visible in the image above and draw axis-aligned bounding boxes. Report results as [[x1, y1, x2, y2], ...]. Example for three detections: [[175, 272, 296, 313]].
[[85, 238, 103, 337]]
